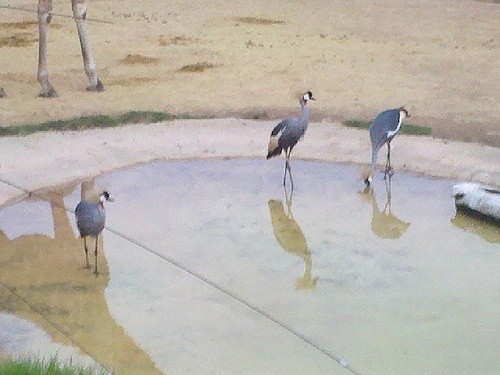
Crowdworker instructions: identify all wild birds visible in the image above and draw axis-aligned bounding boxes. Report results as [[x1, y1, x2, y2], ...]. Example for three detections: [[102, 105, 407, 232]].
[[356, 176, 411, 240], [265, 91, 316, 187], [450, 182, 500, 224], [268, 188, 319, 290], [74, 191, 116, 277], [359, 106, 412, 187]]
